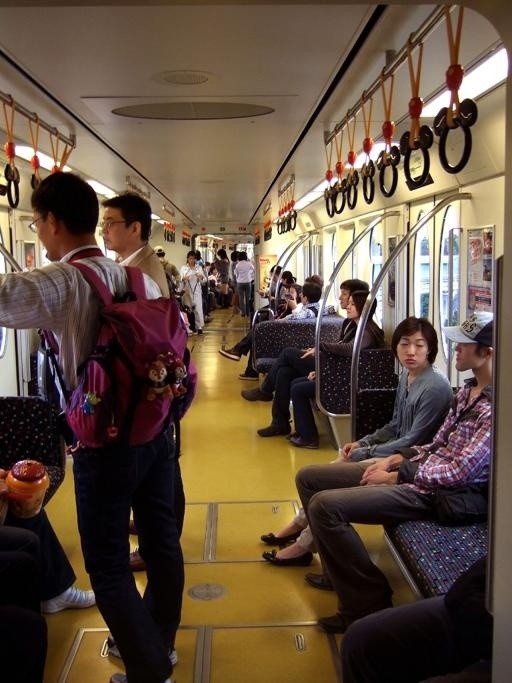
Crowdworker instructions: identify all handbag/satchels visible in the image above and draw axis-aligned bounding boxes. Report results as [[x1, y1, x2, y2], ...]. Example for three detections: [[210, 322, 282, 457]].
[[435, 484, 489, 525]]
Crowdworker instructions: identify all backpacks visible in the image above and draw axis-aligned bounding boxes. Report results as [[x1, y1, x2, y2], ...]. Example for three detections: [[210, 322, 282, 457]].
[[40, 261, 197, 448]]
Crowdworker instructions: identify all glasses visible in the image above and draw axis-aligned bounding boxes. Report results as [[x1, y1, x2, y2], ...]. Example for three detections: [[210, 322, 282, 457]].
[[100, 221, 125, 228], [28, 218, 42, 232]]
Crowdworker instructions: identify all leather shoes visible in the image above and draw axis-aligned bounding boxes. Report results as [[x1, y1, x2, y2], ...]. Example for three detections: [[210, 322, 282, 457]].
[[261, 531, 303, 545], [305, 573, 336, 590], [319, 612, 353, 632], [262, 550, 313, 566]]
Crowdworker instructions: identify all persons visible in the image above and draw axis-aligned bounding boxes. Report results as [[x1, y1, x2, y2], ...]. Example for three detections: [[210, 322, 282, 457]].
[[0, 170, 186, 683], [294, 312, 494, 634], [0, 468, 101, 683], [4, 502, 98, 616], [339, 555, 494, 681], [155, 242, 254, 335], [258, 310, 454, 569], [100, 190, 174, 574], [220, 265, 384, 448]]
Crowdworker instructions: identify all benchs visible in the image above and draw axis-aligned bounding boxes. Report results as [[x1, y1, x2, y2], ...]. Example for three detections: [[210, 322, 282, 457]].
[[253, 312, 395, 452], [1, 395, 65, 507], [354, 386, 489, 599]]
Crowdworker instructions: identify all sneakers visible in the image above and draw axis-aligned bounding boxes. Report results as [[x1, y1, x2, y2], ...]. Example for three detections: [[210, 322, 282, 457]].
[[218, 348, 319, 449], [41, 520, 177, 683]]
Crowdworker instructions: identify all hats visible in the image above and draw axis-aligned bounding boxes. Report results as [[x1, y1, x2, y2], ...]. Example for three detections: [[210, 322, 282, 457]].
[[154, 246, 165, 253], [441, 312, 493, 344]]
